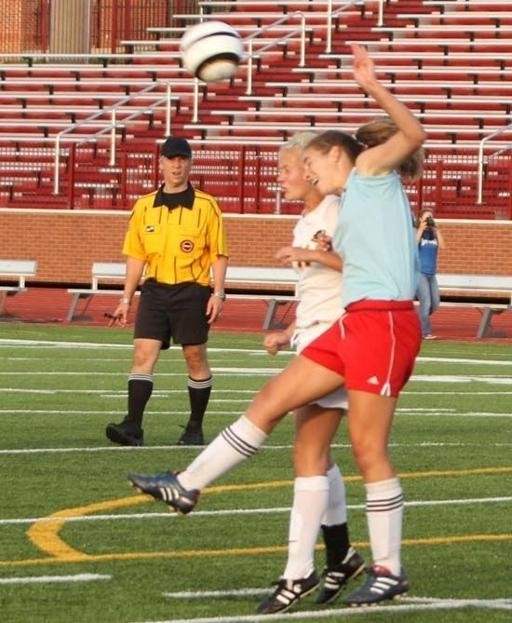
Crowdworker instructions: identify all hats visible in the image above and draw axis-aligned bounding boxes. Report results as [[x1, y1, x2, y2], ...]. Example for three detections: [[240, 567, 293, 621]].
[[162, 137, 191, 159]]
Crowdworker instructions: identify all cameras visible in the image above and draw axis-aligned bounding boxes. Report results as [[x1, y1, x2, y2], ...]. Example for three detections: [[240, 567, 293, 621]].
[[426, 216, 434, 226]]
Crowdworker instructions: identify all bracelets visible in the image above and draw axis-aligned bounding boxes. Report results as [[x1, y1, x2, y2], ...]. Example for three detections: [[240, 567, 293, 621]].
[[213, 291, 226, 301], [120, 298, 131, 304]]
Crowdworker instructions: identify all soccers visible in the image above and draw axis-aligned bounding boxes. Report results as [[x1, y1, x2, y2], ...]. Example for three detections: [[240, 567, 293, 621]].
[[181, 22, 245, 84]]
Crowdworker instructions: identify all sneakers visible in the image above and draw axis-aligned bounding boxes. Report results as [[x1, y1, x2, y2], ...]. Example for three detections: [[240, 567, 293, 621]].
[[127, 471, 200, 514], [258, 546, 409, 614], [177, 421, 203, 445], [106, 420, 144, 446]]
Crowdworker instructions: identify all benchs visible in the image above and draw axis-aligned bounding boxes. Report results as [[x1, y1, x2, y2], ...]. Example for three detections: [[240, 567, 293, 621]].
[[0, 254, 37, 320], [67, 250, 511, 343], [0, 0, 512, 216]]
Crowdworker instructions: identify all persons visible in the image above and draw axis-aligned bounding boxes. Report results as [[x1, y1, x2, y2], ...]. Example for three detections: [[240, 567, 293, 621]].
[[257, 131, 366, 614], [105, 136, 231, 446], [414, 208, 446, 340], [121, 42, 430, 611]]
